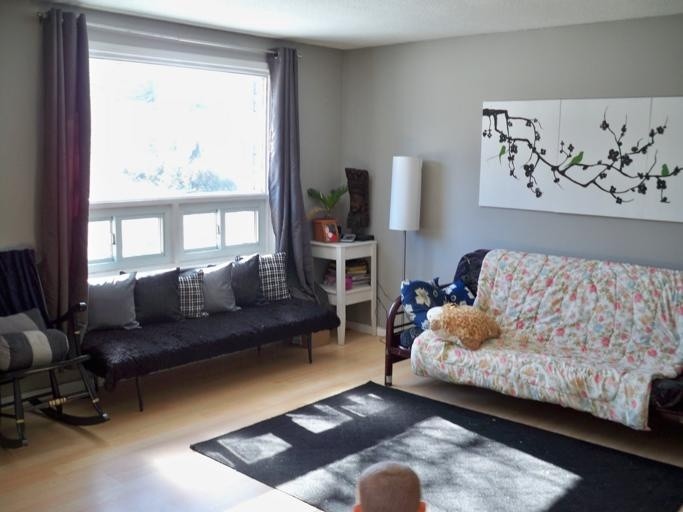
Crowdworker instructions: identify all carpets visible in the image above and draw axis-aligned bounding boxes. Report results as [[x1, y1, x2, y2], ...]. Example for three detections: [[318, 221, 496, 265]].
[[188, 379, 683, 512]]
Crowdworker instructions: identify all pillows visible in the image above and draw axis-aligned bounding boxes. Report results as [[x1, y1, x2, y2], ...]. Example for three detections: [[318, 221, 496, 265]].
[[400, 279, 477, 328], [84, 270, 142, 333], [207, 254, 267, 308], [0, 308, 46, 337], [426, 303, 502, 351], [196, 262, 242, 312], [235, 252, 293, 304], [178, 270, 209, 320], [0, 329, 69, 373], [119, 267, 184, 324]]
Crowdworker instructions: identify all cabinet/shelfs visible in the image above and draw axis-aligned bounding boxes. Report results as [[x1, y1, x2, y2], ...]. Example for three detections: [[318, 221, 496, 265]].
[[310, 240, 378, 345]]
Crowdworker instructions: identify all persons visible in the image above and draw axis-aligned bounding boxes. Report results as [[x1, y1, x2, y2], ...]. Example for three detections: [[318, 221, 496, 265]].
[[352, 461, 426, 512]]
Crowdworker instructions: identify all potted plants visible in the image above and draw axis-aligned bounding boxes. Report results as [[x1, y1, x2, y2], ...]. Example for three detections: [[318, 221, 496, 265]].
[[306, 186, 350, 243]]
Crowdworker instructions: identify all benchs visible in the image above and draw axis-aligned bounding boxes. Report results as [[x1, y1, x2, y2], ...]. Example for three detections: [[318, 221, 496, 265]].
[[78, 296, 337, 413]]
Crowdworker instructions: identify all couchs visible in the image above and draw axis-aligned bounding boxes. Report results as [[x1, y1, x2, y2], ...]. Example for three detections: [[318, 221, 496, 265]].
[[384, 248, 683, 426]]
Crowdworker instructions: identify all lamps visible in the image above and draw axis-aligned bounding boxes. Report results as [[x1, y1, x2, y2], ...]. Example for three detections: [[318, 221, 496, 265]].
[[387, 156, 423, 281]]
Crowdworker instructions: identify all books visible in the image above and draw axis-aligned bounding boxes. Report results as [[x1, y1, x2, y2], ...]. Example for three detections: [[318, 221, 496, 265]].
[[325, 261, 371, 283]]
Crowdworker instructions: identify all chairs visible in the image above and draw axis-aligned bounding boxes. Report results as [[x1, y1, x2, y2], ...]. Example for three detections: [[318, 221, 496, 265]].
[[0, 247, 112, 449]]
